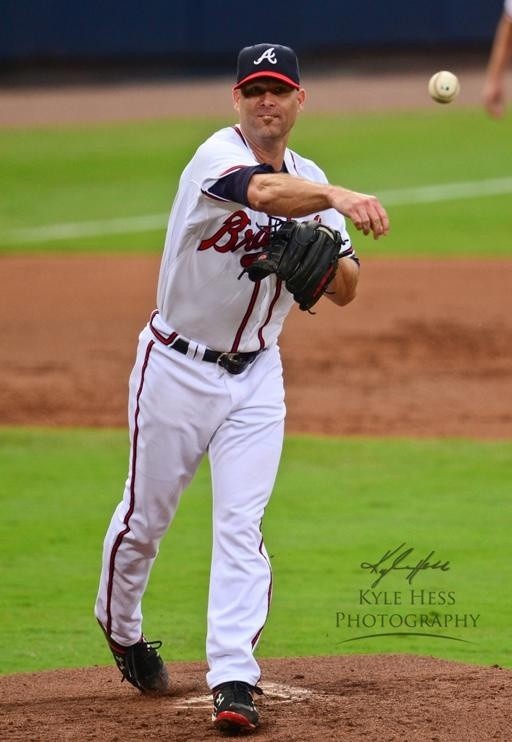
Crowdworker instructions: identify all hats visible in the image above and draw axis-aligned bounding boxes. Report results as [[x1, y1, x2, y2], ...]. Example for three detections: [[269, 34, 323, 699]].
[[231, 43, 302, 91]]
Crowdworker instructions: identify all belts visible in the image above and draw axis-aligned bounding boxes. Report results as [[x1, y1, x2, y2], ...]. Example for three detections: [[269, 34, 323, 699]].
[[170, 338, 269, 374]]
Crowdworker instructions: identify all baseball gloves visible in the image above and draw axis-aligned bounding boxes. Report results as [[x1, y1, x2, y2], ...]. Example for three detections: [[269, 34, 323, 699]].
[[237, 214, 349, 316]]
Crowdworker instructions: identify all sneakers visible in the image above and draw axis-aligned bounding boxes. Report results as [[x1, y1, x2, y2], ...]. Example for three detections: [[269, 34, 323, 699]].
[[96, 616, 169, 696], [211, 681, 259, 732]]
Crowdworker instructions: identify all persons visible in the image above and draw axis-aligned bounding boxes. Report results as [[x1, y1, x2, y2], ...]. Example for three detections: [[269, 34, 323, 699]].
[[481, 0, 512, 122], [92, 39, 389, 734]]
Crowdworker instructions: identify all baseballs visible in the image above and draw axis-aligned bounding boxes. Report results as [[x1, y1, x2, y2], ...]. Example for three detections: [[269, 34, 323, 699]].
[[427, 71, 460, 104]]
